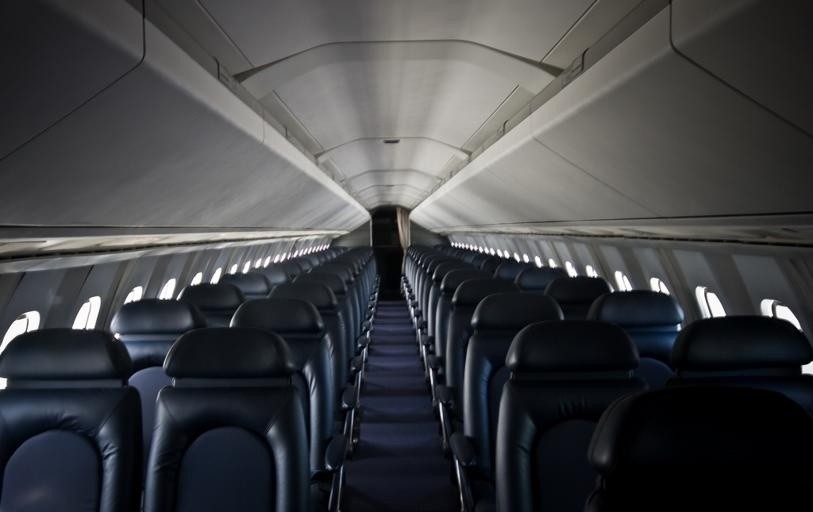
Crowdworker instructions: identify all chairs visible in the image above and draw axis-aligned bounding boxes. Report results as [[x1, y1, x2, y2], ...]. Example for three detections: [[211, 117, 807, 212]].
[[448, 291, 566, 510], [492, 318, 652, 510], [397, 242, 684, 460], [583, 381, 810, 510], [113, 246, 380, 482], [664, 312, 808, 410], [145, 327, 313, 511], [228, 298, 349, 512], [2, 328, 144, 512]]
